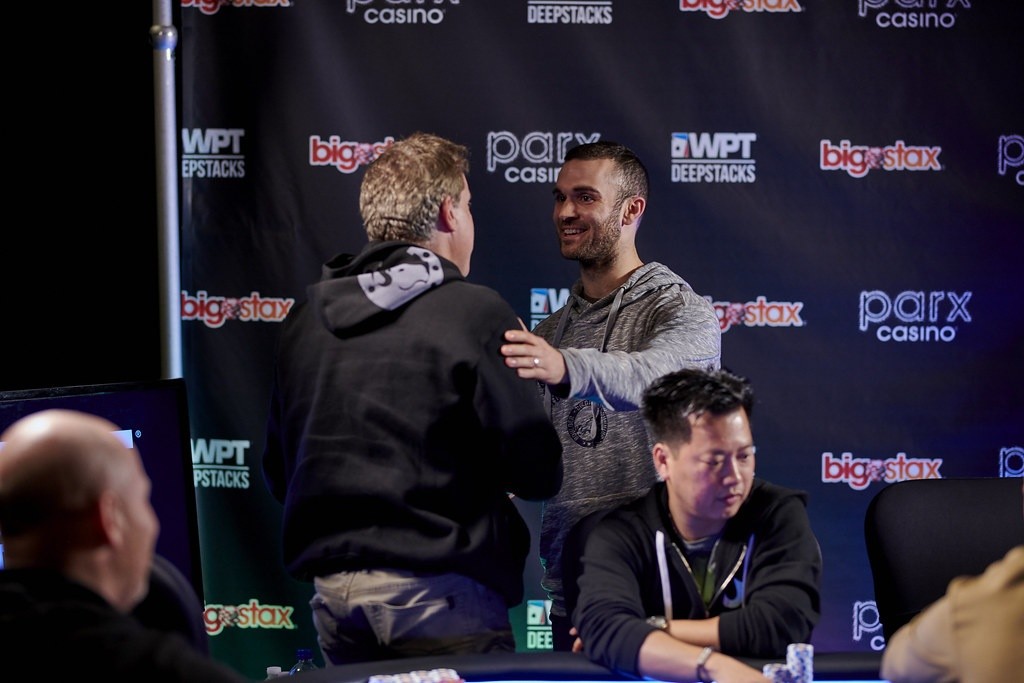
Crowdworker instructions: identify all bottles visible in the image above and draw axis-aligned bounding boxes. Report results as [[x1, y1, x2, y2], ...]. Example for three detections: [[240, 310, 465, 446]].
[[288, 649, 319, 676], [264, 667, 282, 681]]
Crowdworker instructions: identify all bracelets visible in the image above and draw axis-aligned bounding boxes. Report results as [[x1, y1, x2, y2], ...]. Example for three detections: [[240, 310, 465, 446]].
[[696, 645, 720, 683]]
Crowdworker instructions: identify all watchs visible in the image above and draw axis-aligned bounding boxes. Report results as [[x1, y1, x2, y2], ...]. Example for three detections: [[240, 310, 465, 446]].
[[646, 615, 670, 633]]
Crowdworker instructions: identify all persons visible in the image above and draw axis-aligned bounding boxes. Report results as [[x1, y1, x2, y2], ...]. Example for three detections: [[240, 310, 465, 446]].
[[261, 134, 564, 666], [0, 408, 236, 682], [502, 141, 722, 652], [880, 547, 1024, 683], [569, 368, 824, 683]]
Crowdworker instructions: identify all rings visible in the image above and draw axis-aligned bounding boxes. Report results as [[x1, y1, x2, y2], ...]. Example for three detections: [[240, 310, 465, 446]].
[[534, 356, 539, 367]]
